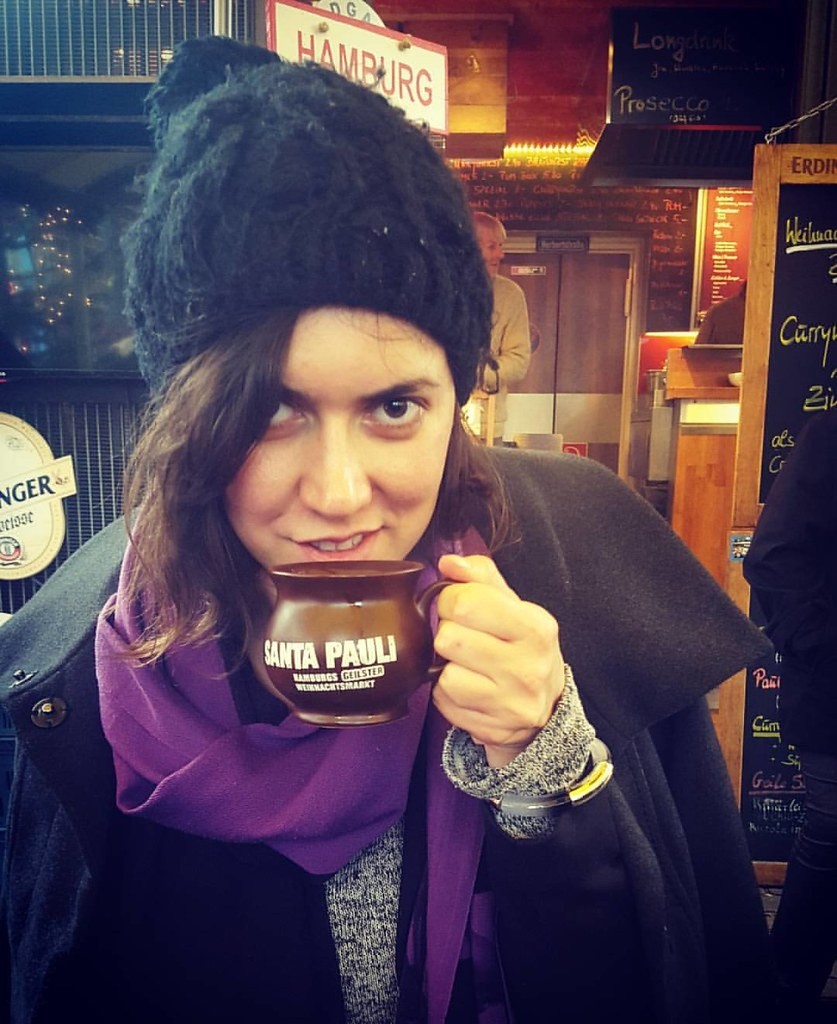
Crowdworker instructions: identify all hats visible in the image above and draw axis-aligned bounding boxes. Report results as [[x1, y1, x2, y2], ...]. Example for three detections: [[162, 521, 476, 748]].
[[125, 34, 495, 403]]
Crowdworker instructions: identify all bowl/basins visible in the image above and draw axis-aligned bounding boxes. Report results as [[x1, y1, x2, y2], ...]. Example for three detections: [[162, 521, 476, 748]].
[[728, 371, 742, 388]]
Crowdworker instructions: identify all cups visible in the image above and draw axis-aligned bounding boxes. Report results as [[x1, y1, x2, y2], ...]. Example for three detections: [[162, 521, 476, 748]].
[[645, 369, 666, 392], [262, 558, 458, 731]]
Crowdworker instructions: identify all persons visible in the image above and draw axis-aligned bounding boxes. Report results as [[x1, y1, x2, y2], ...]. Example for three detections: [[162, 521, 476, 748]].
[[691, 278, 747, 344], [467, 211, 532, 446], [0, 34, 772, 1024], [742, 403, 837, 1024]]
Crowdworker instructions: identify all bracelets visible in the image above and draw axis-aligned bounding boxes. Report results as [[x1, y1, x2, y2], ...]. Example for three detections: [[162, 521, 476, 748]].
[[490, 735, 614, 818]]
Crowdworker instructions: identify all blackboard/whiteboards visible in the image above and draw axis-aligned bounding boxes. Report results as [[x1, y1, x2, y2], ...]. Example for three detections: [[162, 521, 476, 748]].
[[605, 6, 807, 125], [722, 529, 808, 887], [732, 142, 837, 529]]
